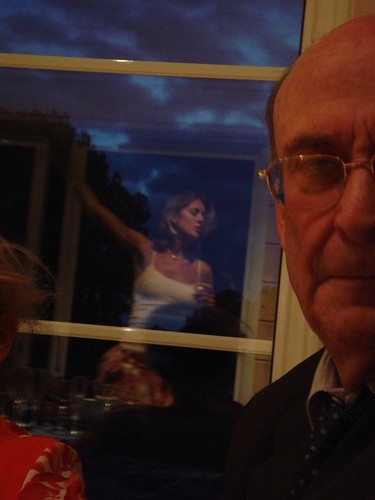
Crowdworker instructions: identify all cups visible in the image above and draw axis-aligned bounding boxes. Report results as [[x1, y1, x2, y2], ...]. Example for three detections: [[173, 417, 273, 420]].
[[4, 363, 121, 437]]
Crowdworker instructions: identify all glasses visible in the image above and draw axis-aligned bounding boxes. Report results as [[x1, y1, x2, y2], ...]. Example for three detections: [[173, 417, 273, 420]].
[[258, 155, 375, 209]]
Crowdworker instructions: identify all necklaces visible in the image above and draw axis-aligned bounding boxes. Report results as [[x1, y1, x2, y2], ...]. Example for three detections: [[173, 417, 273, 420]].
[[167, 248, 186, 259]]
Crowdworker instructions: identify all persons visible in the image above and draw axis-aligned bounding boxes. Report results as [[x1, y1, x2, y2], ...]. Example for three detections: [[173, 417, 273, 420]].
[[78, 182, 217, 403], [231, 11, 374, 499], [0, 237, 85, 500], [71, 306, 243, 499]]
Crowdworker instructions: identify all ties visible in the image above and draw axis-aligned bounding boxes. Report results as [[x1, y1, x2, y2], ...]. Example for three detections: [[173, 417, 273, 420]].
[[291, 393, 355, 500]]
